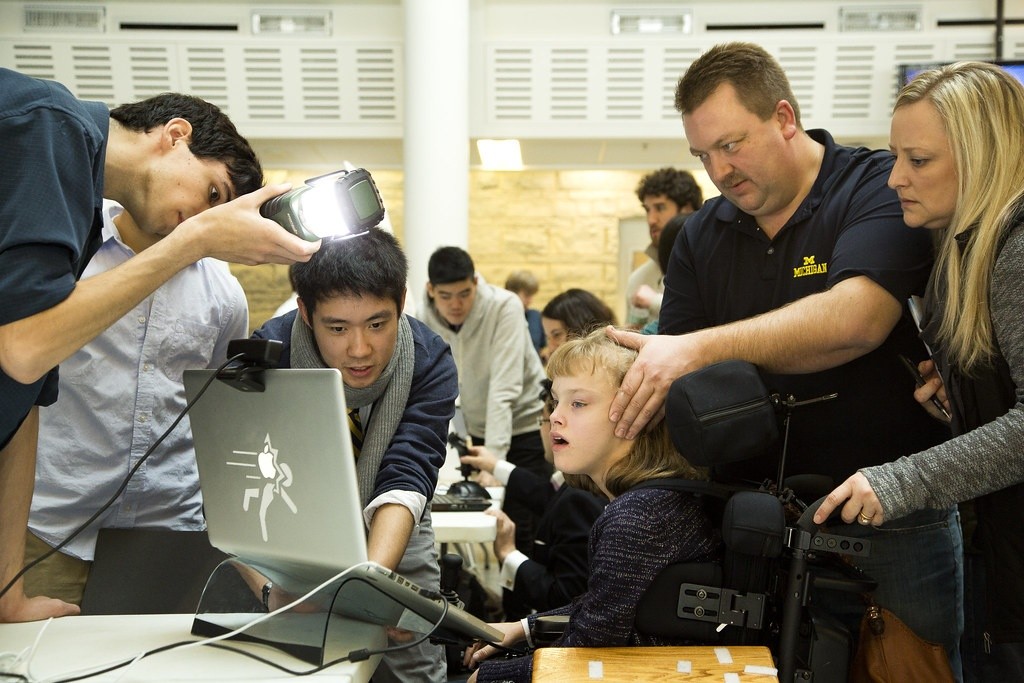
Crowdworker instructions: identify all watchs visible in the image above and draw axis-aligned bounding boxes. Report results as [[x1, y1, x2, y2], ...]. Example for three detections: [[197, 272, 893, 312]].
[[262, 583, 272, 611]]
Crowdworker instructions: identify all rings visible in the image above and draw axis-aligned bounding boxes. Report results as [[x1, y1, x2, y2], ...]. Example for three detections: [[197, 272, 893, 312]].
[[860, 512, 873, 524]]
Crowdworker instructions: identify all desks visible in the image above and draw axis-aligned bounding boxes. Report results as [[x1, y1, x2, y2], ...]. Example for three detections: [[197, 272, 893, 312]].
[[430, 483, 505, 573], [531, 646, 779, 683], [0, 612, 388, 683]]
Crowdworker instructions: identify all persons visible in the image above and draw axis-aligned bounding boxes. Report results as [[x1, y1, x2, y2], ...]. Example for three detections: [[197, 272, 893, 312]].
[[411, 168, 736, 683], [605, 43, 963, 683], [812, 59, 1024, 683], [201, 228, 459, 683], [23, 205, 251, 607], [1, 64, 322, 622]]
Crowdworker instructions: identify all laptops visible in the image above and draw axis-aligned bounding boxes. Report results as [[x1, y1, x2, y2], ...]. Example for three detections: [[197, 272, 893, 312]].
[[181, 367, 505, 645], [430, 492, 491, 512]]
